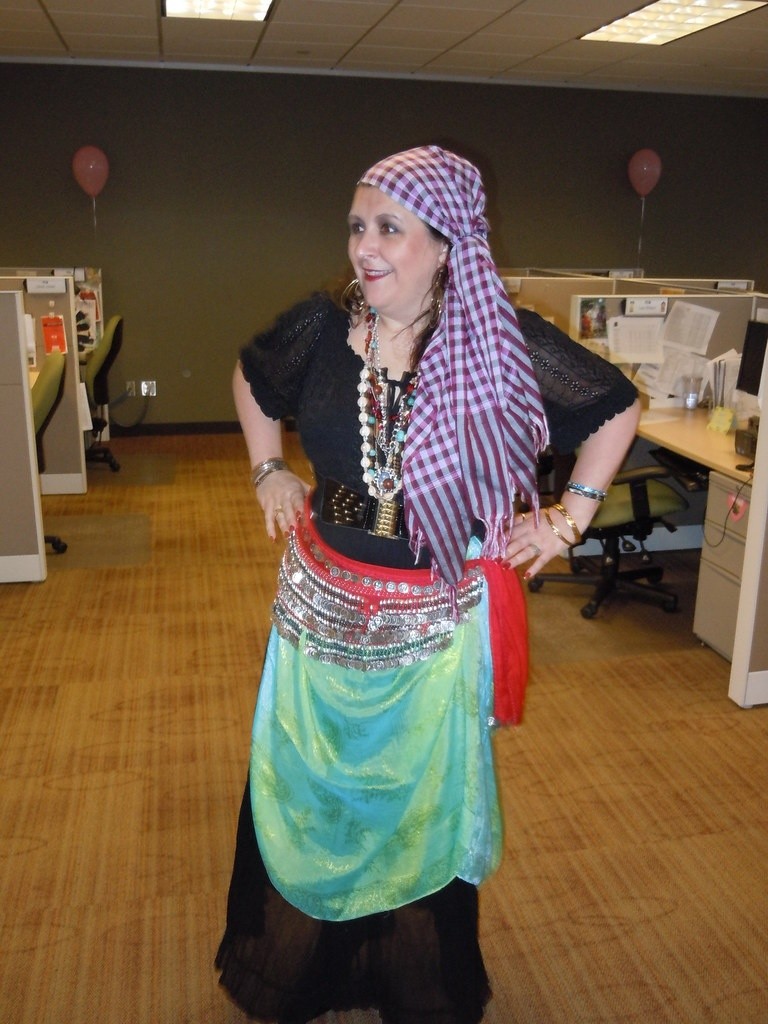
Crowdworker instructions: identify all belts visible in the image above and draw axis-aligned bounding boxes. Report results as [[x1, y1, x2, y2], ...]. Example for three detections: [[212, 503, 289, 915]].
[[312, 471, 415, 540]]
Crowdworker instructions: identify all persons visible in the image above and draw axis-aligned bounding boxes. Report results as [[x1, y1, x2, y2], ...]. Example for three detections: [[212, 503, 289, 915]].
[[208, 145, 648, 1024]]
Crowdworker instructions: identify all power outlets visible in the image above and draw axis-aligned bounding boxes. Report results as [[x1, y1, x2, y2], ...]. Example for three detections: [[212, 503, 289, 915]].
[[126, 381, 135, 397], [141, 381, 156, 396]]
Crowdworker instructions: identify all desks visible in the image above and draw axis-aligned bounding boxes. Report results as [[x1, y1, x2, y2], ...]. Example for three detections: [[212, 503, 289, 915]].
[[30, 370, 40, 389], [635, 406, 760, 666]]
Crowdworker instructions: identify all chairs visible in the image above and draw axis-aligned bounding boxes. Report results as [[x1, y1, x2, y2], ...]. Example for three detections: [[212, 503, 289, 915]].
[[31, 352, 67, 553], [79, 314, 124, 472], [528, 440, 690, 619]]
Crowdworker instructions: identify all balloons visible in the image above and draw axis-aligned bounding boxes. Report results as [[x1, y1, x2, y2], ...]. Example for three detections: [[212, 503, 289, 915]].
[[69, 144, 112, 200], [625, 146, 664, 198]]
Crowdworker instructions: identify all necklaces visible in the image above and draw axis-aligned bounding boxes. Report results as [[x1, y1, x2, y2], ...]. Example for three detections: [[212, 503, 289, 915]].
[[357, 305, 422, 501]]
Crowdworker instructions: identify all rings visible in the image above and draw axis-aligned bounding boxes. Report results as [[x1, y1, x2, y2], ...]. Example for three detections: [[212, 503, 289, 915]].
[[274, 507, 283, 517], [521, 512, 526, 524]]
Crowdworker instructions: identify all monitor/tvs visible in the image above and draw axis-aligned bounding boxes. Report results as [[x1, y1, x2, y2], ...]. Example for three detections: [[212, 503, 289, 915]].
[[737, 320, 768, 396]]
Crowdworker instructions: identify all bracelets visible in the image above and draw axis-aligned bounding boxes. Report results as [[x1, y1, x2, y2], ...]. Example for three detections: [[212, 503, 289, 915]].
[[248, 456, 294, 486], [565, 481, 608, 501], [544, 503, 582, 546]]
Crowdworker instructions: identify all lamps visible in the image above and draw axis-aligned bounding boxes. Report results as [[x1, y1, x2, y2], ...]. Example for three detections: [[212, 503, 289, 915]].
[[580, 0, 768, 45], [166, 0, 272, 22]]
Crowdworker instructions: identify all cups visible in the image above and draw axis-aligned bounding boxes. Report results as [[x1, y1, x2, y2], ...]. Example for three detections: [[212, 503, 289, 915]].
[[682, 376, 703, 410]]
